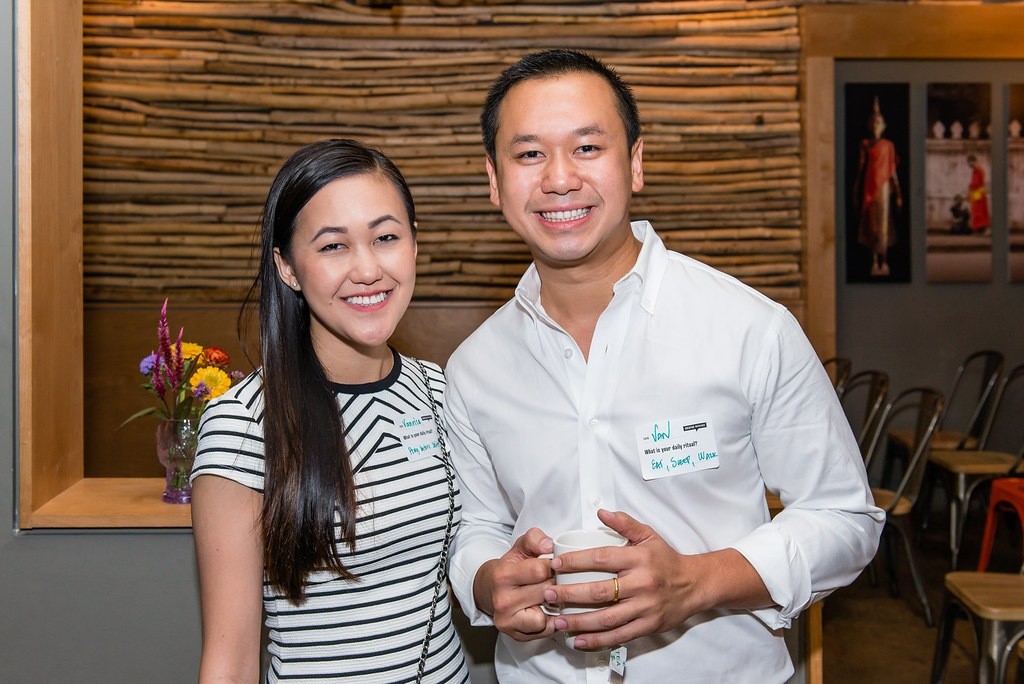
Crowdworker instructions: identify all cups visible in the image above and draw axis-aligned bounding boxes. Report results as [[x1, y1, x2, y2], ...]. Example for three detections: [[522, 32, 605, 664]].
[[538, 531, 631, 655]]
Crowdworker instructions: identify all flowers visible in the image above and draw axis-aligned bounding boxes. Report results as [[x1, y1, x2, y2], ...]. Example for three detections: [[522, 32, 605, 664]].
[[115, 297, 244, 429]]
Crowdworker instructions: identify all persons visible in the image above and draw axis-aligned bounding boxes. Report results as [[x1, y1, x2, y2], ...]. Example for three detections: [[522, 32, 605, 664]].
[[189, 139, 475, 684], [443, 48, 888, 684], [860, 112, 902, 274], [964, 153, 989, 229]]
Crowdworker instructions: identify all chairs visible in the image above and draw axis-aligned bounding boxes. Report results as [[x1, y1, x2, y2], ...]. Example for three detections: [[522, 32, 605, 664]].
[[810, 349, 1023, 684]]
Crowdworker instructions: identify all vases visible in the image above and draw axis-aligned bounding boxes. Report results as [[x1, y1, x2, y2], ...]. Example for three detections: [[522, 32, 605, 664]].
[[154, 409, 202, 505]]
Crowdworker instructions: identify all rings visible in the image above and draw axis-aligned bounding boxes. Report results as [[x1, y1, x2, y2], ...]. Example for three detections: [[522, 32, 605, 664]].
[[614, 578, 619, 602]]
